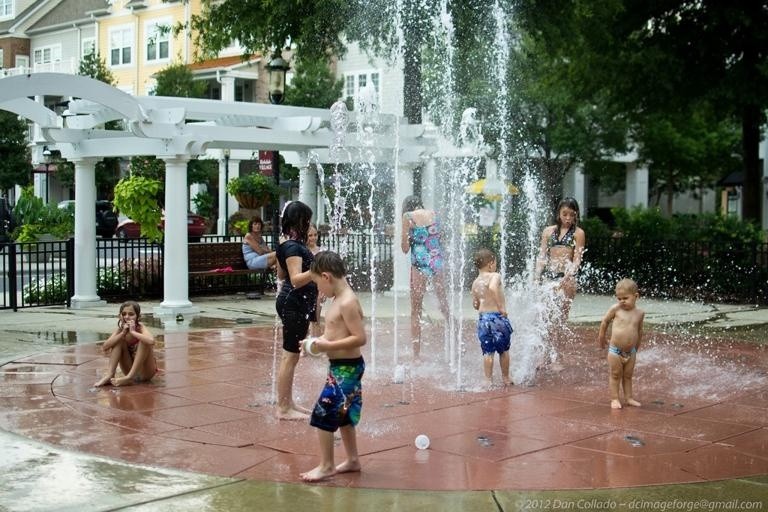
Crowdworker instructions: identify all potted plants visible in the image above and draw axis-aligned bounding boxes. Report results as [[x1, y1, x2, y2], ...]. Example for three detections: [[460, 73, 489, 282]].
[[227, 172, 288, 209]]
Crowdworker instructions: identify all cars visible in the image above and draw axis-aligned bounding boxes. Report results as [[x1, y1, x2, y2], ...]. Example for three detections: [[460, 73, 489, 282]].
[[117, 212, 210, 243]]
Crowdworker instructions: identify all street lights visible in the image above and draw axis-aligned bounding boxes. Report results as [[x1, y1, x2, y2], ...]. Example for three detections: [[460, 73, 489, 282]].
[[266, 47, 289, 251]]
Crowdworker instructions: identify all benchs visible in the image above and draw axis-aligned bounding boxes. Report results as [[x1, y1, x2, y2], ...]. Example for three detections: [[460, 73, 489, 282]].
[[188, 242, 271, 299]]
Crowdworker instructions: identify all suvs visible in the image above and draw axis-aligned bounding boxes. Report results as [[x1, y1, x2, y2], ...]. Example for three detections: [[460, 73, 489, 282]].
[[52, 197, 118, 239]]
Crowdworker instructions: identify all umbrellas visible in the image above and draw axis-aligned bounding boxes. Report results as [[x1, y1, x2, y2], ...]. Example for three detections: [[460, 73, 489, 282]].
[[468, 176, 519, 201]]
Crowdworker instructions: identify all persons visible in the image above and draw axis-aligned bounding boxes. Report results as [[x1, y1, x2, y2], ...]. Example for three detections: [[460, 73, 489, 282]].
[[242, 216, 277, 272], [93, 301, 159, 389], [299, 224, 327, 357], [470, 248, 515, 386], [401, 194, 456, 364], [534, 197, 586, 373], [274, 200, 325, 421], [296, 249, 367, 482], [597, 277, 647, 410]]
